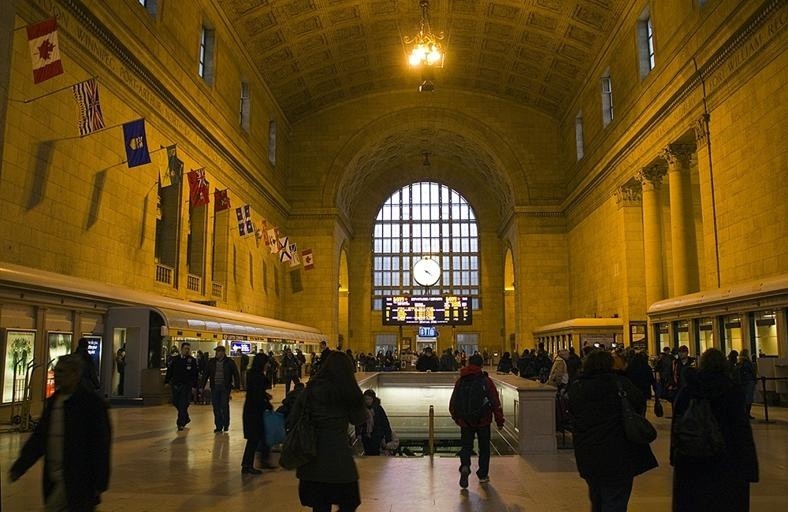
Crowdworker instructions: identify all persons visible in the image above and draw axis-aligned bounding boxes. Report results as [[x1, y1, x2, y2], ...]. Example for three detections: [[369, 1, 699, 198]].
[[166, 342, 248, 433], [550, 341, 759, 512], [415, 342, 557, 383], [260, 341, 400, 397], [288, 352, 370, 512], [116, 348, 125, 396], [8, 342, 113, 511], [354, 389, 399, 456], [449, 355, 505, 489], [241, 353, 279, 475]]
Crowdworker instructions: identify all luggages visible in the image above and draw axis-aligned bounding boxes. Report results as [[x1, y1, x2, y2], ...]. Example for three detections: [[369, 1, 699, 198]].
[[263, 411, 287, 447]]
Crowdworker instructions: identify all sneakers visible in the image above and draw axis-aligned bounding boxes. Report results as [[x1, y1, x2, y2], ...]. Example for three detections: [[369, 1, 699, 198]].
[[240, 467, 261, 475], [480, 476, 489, 483], [214, 427, 220, 432], [178, 426, 184, 430], [460, 468, 468, 487], [186, 418, 191, 424], [224, 427, 229, 431]]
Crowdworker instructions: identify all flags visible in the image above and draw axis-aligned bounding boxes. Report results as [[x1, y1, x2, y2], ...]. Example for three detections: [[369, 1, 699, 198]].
[[157, 144, 183, 188], [70, 77, 105, 137], [214, 190, 315, 271], [26, 16, 64, 85], [187, 168, 210, 208], [122, 117, 151, 168]]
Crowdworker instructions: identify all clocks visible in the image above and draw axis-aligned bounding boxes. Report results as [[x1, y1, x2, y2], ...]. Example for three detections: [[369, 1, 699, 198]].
[[412, 258, 441, 287]]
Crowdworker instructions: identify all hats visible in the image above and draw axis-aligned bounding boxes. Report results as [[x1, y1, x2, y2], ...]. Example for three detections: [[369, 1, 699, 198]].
[[213, 346, 225, 352], [678, 345, 688, 352]]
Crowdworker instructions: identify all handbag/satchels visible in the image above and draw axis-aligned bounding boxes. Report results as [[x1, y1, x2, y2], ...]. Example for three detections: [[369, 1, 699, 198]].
[[279, 416, 311, 472], [654, 402, 664, 417], [623, 412, 657, 449]]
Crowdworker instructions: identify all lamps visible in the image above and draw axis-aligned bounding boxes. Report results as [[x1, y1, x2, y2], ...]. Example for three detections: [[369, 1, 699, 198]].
[[401, 0, 447, 93]]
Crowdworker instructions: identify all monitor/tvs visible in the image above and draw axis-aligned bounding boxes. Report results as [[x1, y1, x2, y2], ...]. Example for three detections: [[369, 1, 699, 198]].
[[419, 326, 437, 337], [232, 343, 250, 352]]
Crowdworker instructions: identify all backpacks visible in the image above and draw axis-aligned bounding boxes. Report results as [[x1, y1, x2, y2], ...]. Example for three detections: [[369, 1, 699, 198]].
[[454, 377, 486, 421]]
